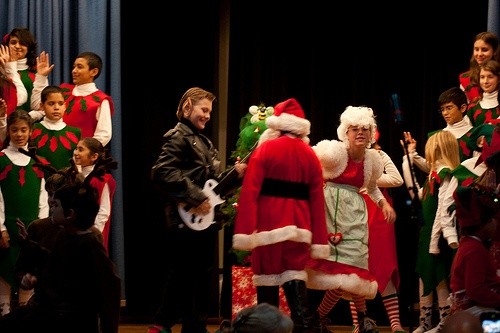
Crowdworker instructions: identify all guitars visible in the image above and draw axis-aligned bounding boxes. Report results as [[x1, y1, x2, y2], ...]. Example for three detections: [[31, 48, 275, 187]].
[[175, 140, 259, 233]]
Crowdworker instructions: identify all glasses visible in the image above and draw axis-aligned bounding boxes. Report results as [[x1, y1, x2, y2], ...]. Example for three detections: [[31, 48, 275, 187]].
[[438, 105, 457, 114]]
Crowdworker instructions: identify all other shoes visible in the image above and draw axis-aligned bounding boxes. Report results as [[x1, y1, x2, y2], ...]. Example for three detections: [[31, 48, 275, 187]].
[[361, 316, 379, 333], [411, 315, 443, 333]]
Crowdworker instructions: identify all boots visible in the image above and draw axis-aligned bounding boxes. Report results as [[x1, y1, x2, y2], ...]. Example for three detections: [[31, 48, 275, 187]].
[[255, 284, 280, 310], [282, 279, 333, 333]]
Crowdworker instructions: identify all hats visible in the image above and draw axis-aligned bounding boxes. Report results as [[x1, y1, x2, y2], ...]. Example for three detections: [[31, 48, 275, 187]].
[[453, 182, 500, 235], [264, 98, 311, 138], [336, 104, 379, 147]]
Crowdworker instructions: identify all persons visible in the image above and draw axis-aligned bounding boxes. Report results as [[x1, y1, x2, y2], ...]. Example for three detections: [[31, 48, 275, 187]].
[[308, 105, 397, 333], [350, 128, 407, 333], [151, 88, 248, 333], [232, 97, 328, 333], [0, 29, 124, 333], [402, 31, 500, 333]]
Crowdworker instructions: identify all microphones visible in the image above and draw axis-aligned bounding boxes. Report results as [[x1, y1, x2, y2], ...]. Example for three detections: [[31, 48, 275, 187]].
[[389, 93, 402, 124]]
